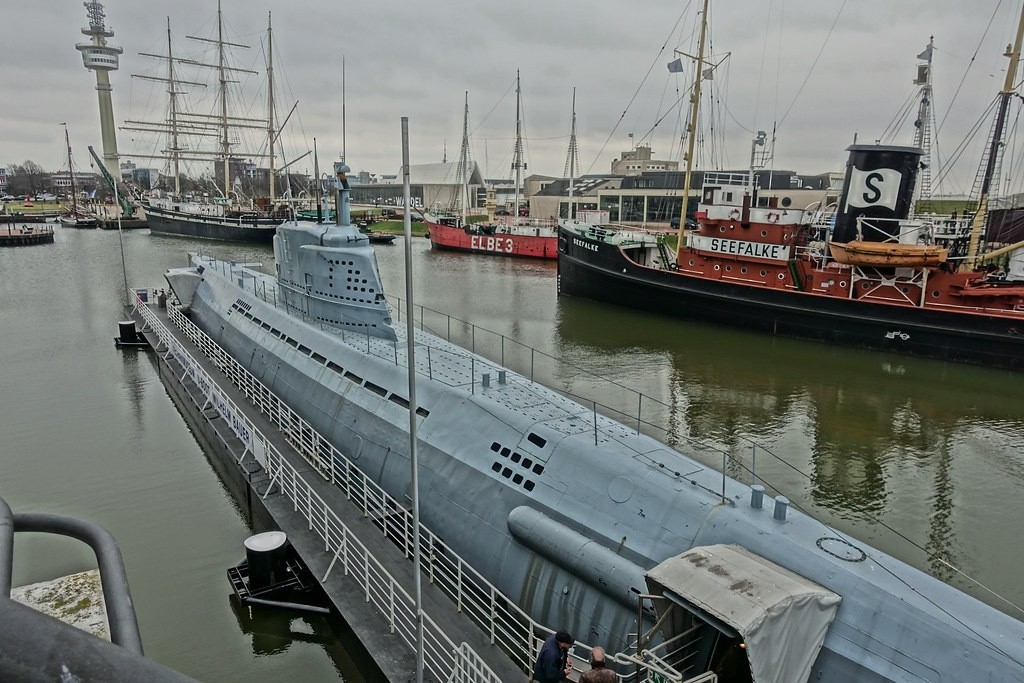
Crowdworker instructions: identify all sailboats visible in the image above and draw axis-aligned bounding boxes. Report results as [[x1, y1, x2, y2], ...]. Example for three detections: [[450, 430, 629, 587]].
[[118, 0, 366, 244], [425, 69, 582, 260], [556, 0, 1023, 372]]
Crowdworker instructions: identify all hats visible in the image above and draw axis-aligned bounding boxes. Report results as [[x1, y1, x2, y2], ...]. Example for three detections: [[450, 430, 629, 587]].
[[555, 629, 574, 644], [590, 646, 606, 661]]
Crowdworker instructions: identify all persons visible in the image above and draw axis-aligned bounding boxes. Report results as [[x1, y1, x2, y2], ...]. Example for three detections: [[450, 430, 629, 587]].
[[533, 629, 573, 683], [579, 646, 619, 683]]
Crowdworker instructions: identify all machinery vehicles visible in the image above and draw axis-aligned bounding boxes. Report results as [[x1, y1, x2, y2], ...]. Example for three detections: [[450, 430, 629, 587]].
[[87, 145, 139, 221]]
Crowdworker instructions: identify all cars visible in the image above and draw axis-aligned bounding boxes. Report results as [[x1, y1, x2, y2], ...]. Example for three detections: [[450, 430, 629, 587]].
[[1, 194, 16, 201], [409, 211, 425, 223], [496, 210, 510, 216], [670, 217, 698, 230], [15, 194, 28, 200], [33, 193, 43, 199]]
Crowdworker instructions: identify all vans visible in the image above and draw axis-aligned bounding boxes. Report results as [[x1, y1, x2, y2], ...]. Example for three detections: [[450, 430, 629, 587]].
[[382, 208, 397, 218]]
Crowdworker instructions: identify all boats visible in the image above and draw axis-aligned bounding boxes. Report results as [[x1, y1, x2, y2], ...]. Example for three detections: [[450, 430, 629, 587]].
[[95, 210, 148, 230], [55, 123, 97, 228], [120, 112, 1024, 683]]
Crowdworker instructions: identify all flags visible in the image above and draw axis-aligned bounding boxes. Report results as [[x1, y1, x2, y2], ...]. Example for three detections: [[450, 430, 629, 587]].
[[667, 59, 683, 72], [702, 69, 712, 80]]
